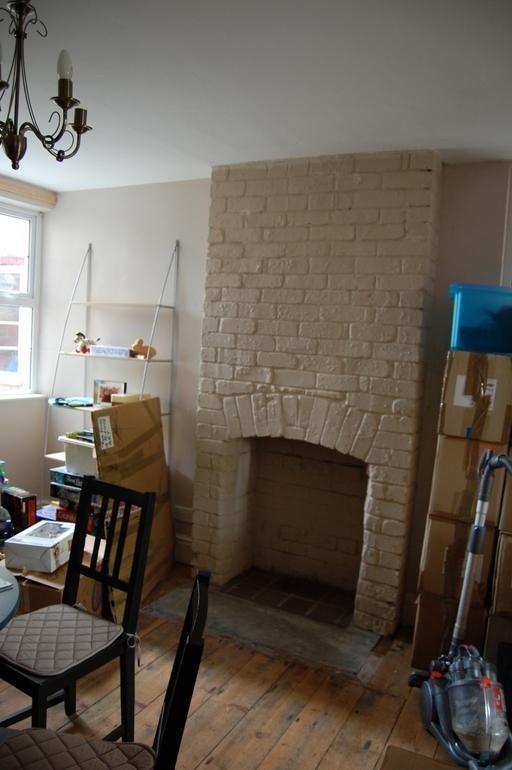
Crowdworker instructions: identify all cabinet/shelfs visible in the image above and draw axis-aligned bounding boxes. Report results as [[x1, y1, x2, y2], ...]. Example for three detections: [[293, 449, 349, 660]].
[[37, 238, 183, 511], [49, 463, 102, 507]]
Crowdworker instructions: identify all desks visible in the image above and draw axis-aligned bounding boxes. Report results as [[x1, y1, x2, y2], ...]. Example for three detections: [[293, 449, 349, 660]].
[[1, 562, 23, 630]]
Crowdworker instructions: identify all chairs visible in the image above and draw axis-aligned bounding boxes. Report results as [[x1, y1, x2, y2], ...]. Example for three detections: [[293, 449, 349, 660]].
[[1, 568, 213, 770], [1, 478, 158, 749]]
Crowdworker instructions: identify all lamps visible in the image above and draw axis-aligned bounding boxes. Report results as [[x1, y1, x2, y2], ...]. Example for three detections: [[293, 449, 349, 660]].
[[0, 0, 95, 169]]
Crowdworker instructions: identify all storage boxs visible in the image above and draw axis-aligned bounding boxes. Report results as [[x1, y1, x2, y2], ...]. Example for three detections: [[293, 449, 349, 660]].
[[5, 520, 75, 570], [428, 435, 503, 530], [498, 467, 511, 536], [417, 516, 497, 606], [481, 613, 509, 687], [445, 279, 509, 356], [436, 352, 510, 444], [5, 533, 110, 621], [411, 593, 489, 673], [58, 431, 100, 478], [490, 532, 511, 615]]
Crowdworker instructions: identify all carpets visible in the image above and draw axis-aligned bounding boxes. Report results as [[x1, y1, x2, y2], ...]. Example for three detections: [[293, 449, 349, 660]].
[[379, 744, 456, 770]]
